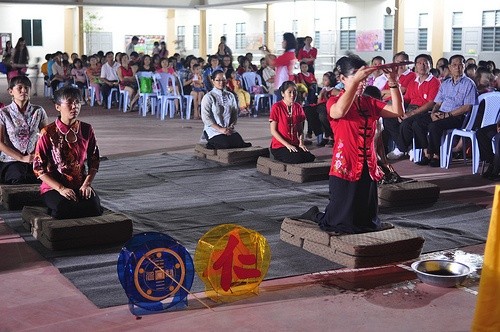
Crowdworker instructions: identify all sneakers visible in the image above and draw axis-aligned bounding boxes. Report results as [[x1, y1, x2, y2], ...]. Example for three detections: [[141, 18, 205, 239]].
[[387, 148, 407, 160]]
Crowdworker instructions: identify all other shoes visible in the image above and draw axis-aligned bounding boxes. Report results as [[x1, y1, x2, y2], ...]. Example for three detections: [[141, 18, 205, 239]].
[[482, 158, 499, 178], [319, 139, 333, 147], [240, 107, 252, 114]]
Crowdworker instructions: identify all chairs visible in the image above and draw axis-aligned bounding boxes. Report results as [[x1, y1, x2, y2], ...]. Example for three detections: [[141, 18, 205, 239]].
[[42, 64, 500, 175]]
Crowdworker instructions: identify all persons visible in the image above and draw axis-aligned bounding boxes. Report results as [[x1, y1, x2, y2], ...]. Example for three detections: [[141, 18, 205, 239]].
[[201, 70, 252, 150], [297, 36, 318, 75], [0, 76, 48, 185], [383, 54, 441, 160], [294, 58, 344, 148], [2, 41, 13, 83], [33, 85, 102, 220], [382, 51, 416, 105], [298, 56, 404, 234], [41, 35, 205, 120], [475, 114, 500, 179], [365, 55, 389, 90], [429, 55, 452, 83], [204, 36, 277, 117], [413, 54, 479, 167], [363, 85, 402, 185], [8, 37, 30, 90], [269, 81, 315, 164], [259, 32, 296, 104], [464, 58, 500, 97]]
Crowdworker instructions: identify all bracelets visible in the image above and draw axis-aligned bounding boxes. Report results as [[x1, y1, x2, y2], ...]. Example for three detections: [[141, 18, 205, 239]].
[[444, 112, 445, 118], [448, 112, 452, 117]]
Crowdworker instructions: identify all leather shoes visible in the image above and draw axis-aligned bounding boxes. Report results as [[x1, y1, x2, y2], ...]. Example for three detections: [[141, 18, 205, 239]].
[[430, 158, 440, 167], [416, 159, 431, 165]]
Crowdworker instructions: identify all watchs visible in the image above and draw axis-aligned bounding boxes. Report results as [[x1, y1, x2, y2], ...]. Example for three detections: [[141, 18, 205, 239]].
[[389, 84, 399, 88]]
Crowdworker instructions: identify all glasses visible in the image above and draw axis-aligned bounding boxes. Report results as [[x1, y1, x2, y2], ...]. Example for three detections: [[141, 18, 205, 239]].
[[216, 79, 227, 83]]
